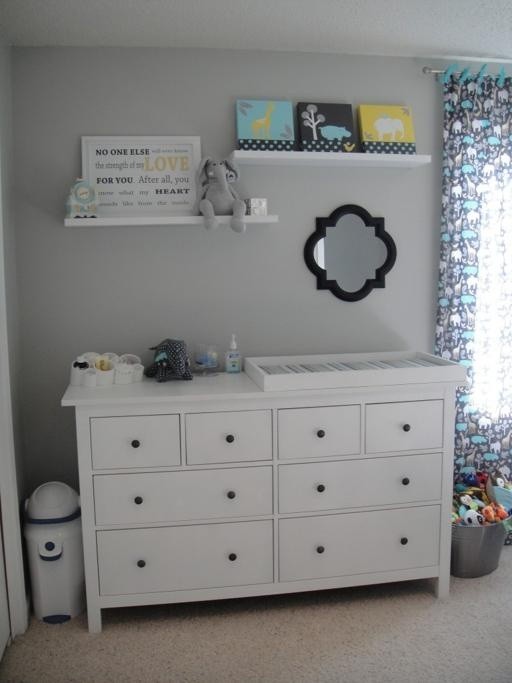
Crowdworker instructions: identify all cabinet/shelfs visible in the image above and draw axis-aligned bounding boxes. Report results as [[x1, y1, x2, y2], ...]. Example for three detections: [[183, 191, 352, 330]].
[[60, 371, 463, 632]]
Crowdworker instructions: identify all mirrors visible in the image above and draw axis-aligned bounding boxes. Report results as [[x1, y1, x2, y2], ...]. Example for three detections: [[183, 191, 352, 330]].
[[301, 204, 399, 301]]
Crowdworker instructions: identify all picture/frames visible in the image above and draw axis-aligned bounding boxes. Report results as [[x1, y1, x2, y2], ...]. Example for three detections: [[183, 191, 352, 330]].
[[83, 136, 204, 220]]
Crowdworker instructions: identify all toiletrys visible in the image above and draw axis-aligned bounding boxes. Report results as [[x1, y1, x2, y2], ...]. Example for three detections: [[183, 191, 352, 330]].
[[225, 334, 242, 373]]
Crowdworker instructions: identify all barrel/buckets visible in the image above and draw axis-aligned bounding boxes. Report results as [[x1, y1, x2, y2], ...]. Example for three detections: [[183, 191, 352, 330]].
[[451, 512, 512, 579]]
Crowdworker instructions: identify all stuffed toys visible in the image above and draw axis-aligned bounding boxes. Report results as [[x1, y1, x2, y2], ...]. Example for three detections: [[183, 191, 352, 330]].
[[144, 337, 193, 382], [452, 468, 512, 526], [189, 155, 247, 234]]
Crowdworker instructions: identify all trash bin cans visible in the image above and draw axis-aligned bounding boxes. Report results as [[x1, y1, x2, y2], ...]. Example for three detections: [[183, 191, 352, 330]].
[[24, 481, 85, 626]]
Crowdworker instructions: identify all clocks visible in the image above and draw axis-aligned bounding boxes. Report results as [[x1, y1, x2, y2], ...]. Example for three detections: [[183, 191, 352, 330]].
[[66, 178, 99, 218]]
